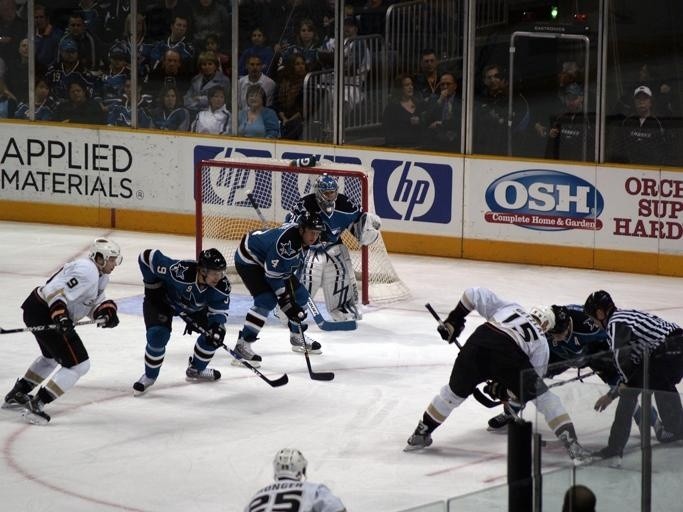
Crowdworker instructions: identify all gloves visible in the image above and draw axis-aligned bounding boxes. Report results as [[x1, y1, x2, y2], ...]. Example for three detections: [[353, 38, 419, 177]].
[[210, 327, 226, 347], [281, 301, 306, 322], [93, 301, 119, 328], [485, 381, 507, 398], [437, 315, 465, 344], [50, 308, 75, 334]]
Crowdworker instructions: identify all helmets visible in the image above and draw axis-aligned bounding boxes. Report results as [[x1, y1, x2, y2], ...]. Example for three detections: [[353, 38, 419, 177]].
[[314, 177, 338, 204], [90, 238, 123, 268], [199, 249, 226, 271], [299, 213, 327, 231], [585, 290, 612, 312], [273, 448, 306, 479], [531, 305, 570, 334]]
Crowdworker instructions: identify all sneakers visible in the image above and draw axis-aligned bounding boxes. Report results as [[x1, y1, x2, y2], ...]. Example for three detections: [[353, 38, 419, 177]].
[[234, 331, 261, 362], [408, 432, 433, 446], [290, 333, 321, 350], [488, 414, 513, 428], [5, 389, 51, 421], [133, 373, 156, 391], [567, 442, 592, 459], [186, 357, 220, 381]]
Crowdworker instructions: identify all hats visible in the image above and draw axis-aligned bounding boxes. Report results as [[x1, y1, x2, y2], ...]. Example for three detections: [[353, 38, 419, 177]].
[[634, 86, 653, 98], [567, 84, 581, 96]]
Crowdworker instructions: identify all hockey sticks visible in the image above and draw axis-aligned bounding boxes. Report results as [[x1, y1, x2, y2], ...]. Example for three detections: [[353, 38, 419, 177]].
[[174, 307, 289, 388], [246, 190, 359, 331], [285, 276, 336, 382], [471, 370, 603, 409], [0, 318, 107, 335]]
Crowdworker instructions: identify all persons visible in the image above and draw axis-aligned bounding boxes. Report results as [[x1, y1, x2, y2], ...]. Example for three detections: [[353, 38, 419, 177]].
[[129, 248, 231, 394], [242, 448, 348, 512], [1, 1, 682, 166], [233, 212, 326, 361], [485, 303, 675, 445], [562, 485, 598, 512], [405, 285, 605, 466], [286, 174, 381, 322], [579, 290, 683, 458], [4, 239, 122, 423]]
